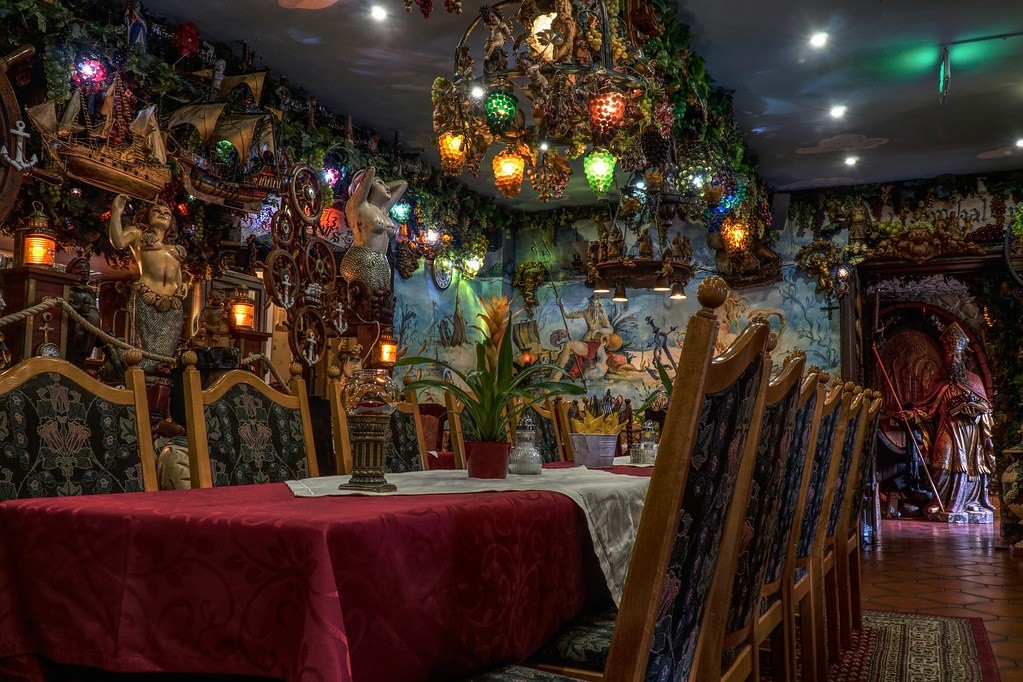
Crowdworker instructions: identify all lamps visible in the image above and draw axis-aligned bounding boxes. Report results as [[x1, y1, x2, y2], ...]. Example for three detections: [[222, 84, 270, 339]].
[[372, 327, 396, 368], [425, 0, 772, 303], [72, 55, 108, 97], [229, 284, 255, 330], [391, 202, 412, 224], [324, 166, 340, 187], [432, 254, 453, 290], [14, 200, 56, 270]]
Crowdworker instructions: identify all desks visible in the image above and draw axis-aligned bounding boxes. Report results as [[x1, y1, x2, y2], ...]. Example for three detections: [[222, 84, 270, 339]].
[[0, 457, 656, 681]]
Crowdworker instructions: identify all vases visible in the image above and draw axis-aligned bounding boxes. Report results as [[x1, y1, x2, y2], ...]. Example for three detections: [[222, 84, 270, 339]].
[[463, 438, 510, 479]]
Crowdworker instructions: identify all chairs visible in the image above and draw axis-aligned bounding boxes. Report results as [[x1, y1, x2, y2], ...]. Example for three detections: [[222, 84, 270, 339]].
[[182, 351, 320, 488], [0, 349, 159, 503], [326, 366, 431, 475], [466, 274, 883, 682], [444, 379, 634, 470]]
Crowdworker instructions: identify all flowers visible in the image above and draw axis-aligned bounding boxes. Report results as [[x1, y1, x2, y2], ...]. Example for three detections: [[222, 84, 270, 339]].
[[388, 292, 589, 443]]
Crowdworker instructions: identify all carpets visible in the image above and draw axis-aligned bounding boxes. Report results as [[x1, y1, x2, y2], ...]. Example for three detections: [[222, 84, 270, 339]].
[[745, 607, 1001, 682]]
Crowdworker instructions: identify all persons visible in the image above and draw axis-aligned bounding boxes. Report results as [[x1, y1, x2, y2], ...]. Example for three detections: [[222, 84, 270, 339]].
[[804, 253, 834, 302], [842, 194, 872, 245], [901, 360, 933, 400], [109, 194, 188, 369], [335, 337, 366, 383], [517, 271, 542, 318], [190, 290, 236, 349], [603, 226, 694, 261], [897, 320, 995, 515], [395, 241, 418, 280], [63, 258, 100, 368], [904, 198, 971, 245], [340, 166, 407, 295]]
